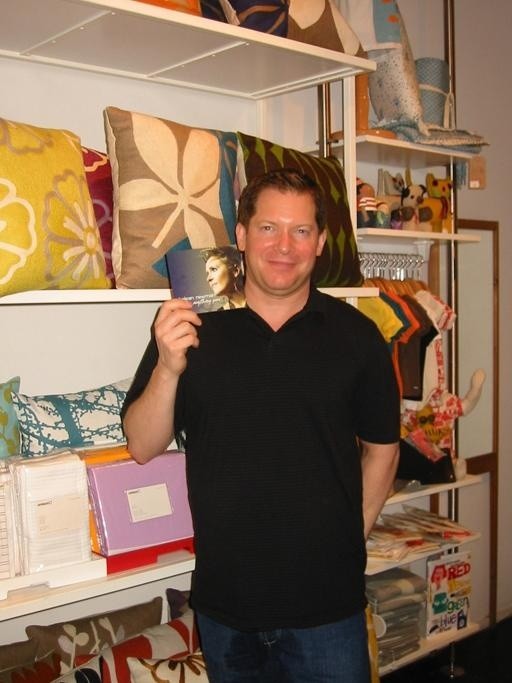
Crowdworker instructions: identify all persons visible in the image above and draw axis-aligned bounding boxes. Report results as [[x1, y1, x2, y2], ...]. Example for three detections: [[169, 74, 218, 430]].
[[119, 169, 401, 682], [200, 246, 247, 312]]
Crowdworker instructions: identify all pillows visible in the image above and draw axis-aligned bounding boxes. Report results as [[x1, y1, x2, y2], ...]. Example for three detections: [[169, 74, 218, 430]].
[[12, 371, 136, 459], [54, 609, 198, 683], [134, 0, 201, 16], [102, 103, 249, 290], [24, 594, 162, 673], [166, 587, 192, 620], [80, 146, 116, 293], [0, 118, 111, 299], [1, 646, 61, 683], [199, 0, 288, 39], [236, 125, 365, 288], [127, 649, 209, 683], [0, 636, 40, 672], [0, 375, 20, 459], [288, 0, 369, 60]]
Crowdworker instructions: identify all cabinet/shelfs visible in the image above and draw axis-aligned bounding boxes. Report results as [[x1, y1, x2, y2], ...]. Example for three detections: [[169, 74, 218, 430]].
[[359, 135, 484, 682], [0, 0, 377, 682]]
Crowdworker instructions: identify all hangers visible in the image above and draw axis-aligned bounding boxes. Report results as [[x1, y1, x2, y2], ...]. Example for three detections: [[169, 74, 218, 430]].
[[353, 252, 441, 303]]
